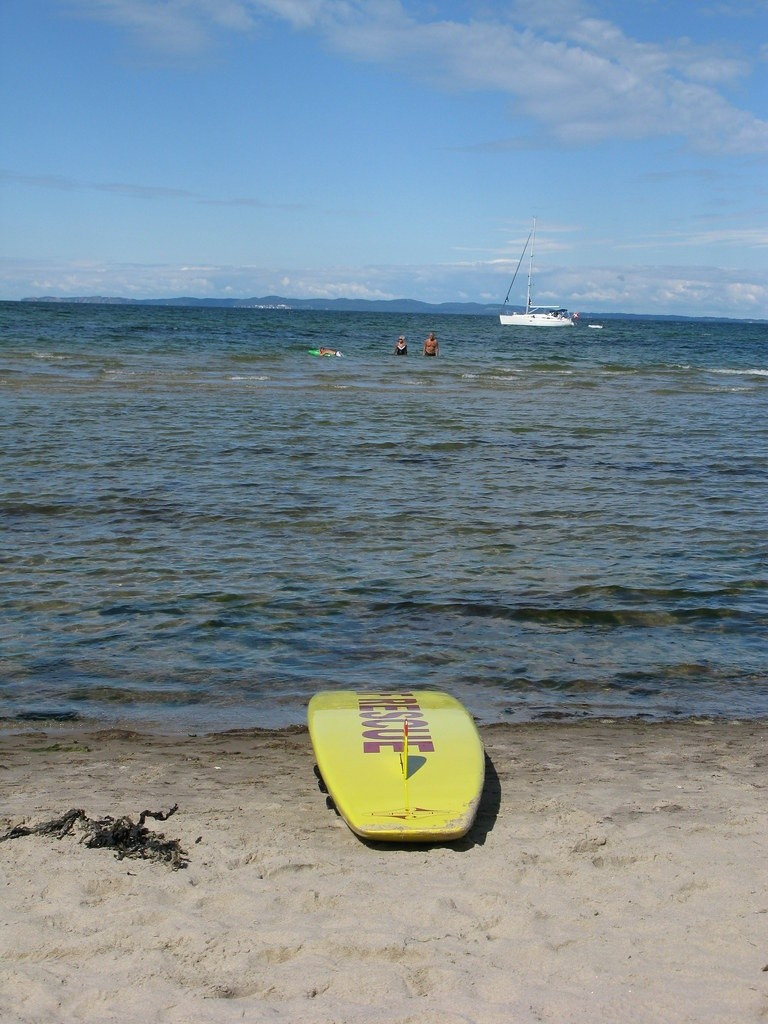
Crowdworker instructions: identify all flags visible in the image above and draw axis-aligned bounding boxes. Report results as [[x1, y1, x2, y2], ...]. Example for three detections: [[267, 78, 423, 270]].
[[572, 312, 579, 320]]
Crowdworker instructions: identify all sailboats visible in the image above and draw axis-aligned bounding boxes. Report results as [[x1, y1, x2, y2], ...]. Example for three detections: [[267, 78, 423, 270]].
[[499, 216, 580, 327]]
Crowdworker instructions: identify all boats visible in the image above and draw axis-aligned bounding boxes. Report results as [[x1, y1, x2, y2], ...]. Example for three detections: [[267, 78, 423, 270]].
[[588, 324, 603, 329]]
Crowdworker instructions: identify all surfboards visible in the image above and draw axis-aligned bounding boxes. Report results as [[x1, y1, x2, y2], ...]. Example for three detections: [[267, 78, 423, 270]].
[[308, 692, 485, 841], [307, 349, 335, 357]]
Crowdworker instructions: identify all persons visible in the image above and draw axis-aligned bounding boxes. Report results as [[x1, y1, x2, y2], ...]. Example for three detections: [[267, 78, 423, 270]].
[[320, 347, 344, 358], [393, 335, 408, 355], [422, 332, 439, 357]]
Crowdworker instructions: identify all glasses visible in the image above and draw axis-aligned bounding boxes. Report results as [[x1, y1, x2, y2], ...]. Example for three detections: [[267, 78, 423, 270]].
[[399, 339, 403, 340]]
